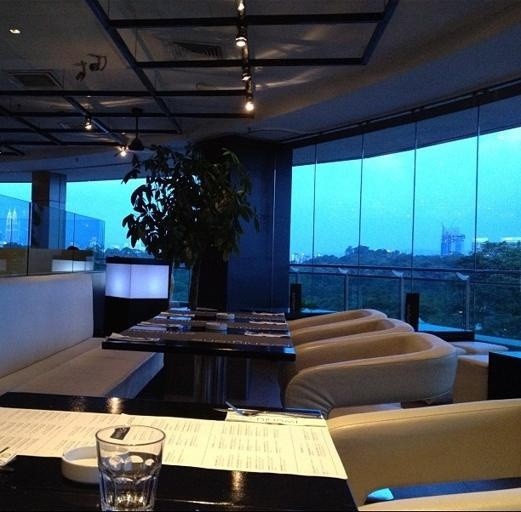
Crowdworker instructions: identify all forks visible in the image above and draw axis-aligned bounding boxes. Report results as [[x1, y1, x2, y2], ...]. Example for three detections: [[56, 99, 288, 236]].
[[224, 400, 322, 418]]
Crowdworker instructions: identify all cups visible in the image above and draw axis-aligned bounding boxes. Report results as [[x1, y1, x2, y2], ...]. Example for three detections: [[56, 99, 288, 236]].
[[95, 425, 166, 512]]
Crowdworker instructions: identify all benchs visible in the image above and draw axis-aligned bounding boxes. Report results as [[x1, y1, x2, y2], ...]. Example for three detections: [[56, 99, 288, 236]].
[[0, 272, 163, 398]]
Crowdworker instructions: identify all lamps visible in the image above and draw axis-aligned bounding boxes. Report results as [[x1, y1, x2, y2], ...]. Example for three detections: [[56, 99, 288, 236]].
[[235, 24, 253, 81]]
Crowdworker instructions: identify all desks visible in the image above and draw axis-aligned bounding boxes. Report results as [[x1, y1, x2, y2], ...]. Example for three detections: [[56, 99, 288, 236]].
[[0, 392, 359, 512], [102, 307, 296, 404]]
